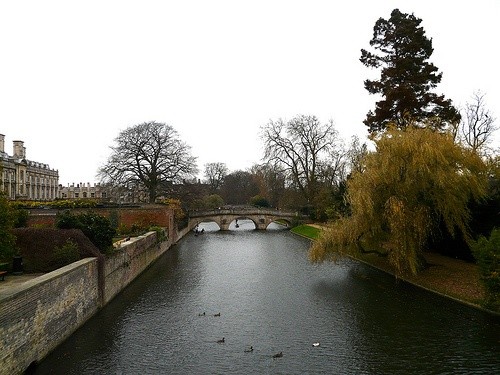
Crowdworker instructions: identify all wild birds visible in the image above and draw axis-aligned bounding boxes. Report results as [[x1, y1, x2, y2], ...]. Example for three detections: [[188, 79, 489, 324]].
[[312, 342, 320, 347], [199, 312, 205, 316], [215, 312, 220, 316], [273, 352, 283, 358], [217, 337, 224, 342], [244, 346, 253, 352]]
